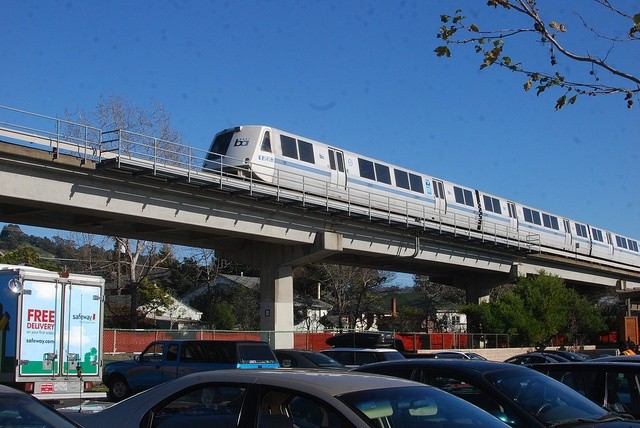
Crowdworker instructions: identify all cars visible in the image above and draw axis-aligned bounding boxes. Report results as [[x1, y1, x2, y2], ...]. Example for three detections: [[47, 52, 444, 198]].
[[57, 368, 511, 428], [0, 384, 86, 428], [431, 352, 486, 360], [349, 359, 640, 428], [504, 355, 561, 385], [273, 349, 347, 370]]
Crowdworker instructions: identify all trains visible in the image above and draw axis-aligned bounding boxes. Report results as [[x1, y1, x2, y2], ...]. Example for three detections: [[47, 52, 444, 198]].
[[202, 125, 640, 272]]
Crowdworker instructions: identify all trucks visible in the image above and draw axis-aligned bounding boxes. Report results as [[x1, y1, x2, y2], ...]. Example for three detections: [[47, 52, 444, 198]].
[[0, 264, 108, 417]]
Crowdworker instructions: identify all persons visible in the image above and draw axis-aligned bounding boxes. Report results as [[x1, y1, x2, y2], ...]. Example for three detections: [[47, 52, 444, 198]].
[[618, 339, 637, 355]]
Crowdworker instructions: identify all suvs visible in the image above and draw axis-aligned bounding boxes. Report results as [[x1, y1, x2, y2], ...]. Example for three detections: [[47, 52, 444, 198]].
[[524, 361, 640, 422], [319, 348, 406, 369], [102, 340, 280, 402]]
